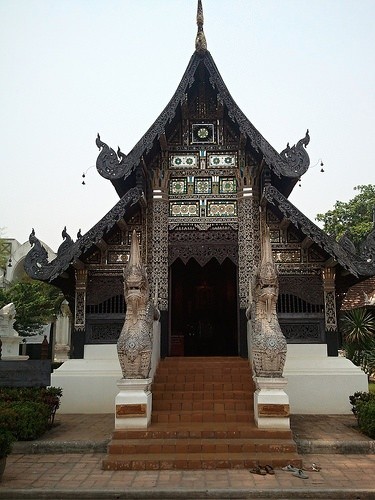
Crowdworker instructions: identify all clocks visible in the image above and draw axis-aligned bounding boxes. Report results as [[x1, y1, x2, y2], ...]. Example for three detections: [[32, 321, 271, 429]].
[[192, 123, 214, 143]]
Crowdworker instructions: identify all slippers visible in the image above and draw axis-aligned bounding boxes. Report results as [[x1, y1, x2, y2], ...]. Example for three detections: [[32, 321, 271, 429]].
[[261, 463, 275, 475], [291, 470, 309, 479], [250, 465, 266, 475], [281, 463, 300, 471]]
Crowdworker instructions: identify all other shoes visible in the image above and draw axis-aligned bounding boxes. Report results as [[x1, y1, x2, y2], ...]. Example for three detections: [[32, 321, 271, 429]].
[[301, 463, 321, 472]]
